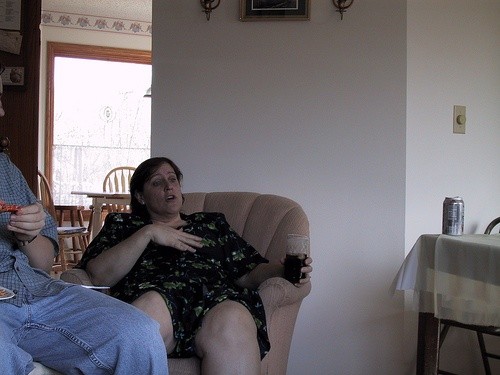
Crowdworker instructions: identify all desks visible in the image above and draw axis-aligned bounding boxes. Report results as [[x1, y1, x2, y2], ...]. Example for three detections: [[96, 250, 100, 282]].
[[71, 190, 132, 257], [388, 230, 500, 375]]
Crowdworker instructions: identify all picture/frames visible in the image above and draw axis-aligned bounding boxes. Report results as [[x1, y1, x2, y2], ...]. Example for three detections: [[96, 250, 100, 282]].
[[239, 0, 312, 22]]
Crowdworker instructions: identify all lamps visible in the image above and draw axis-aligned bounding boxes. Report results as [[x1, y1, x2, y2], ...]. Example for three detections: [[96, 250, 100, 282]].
[[199, 0, 220, 21], [332, 0, 354, 20]]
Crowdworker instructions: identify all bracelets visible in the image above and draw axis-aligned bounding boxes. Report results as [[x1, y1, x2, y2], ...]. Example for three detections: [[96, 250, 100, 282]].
[[14, 235, 38, 247]]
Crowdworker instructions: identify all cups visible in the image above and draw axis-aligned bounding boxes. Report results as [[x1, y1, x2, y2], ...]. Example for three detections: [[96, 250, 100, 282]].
[[283, 233, 310, 285]]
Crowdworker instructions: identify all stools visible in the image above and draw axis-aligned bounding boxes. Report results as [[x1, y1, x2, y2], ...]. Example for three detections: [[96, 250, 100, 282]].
[[54, 202, 89, 266]]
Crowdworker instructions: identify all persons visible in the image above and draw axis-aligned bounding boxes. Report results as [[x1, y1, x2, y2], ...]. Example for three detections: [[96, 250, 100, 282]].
[[0, 63, 169, 375], [80, 157, 313, 375]]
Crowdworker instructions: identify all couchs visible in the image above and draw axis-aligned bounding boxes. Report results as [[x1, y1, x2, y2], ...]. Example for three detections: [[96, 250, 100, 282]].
[[55, 189, 313, 375]]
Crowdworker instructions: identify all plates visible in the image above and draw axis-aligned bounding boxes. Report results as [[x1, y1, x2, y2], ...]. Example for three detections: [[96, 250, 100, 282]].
[[0, 286, 16, 301]]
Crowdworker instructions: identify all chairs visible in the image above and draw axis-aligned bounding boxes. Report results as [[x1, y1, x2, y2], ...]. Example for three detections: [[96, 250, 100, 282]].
[[103, 164, 138, 192], [440, 217, 500, 375], [35, 168, 93, 276]]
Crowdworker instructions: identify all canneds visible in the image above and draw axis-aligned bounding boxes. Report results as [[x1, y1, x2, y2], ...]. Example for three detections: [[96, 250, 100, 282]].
[[442, 196, 465, 236]]
